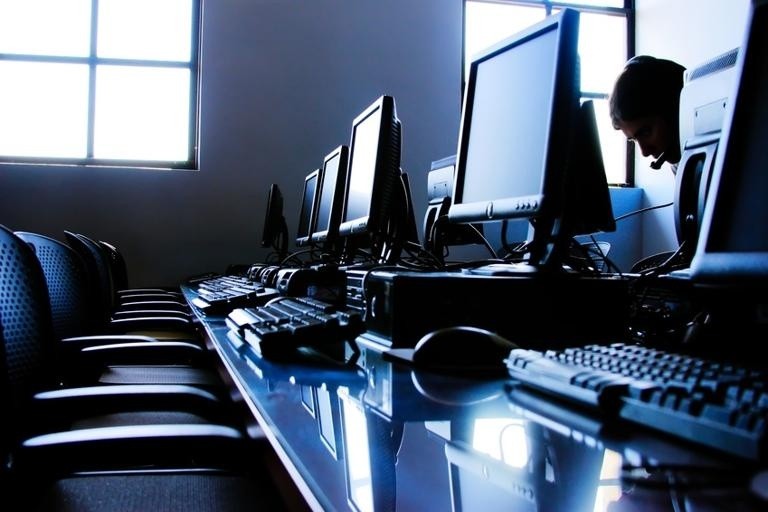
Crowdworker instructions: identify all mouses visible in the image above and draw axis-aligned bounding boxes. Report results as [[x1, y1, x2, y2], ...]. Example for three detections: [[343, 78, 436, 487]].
[[412, 326, 519, 377]]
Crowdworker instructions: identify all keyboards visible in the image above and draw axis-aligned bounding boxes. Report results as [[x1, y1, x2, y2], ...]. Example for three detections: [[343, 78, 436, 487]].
[[186, 274, 364, 359], [502, 341, 767, 464]]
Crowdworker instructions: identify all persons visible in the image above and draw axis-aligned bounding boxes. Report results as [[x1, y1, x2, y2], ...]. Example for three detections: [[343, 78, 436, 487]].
[[609, 55, 688, 177]]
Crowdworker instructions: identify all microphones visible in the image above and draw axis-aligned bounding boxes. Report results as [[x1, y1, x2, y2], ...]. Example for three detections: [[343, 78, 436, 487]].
[[651, 145, 671, 169]]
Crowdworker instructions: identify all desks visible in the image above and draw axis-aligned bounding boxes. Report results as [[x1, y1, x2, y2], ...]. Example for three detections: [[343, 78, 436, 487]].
[[179, 275, 723, 509]]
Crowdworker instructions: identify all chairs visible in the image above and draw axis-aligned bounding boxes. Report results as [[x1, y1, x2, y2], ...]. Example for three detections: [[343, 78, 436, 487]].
[[581, 241, 677, 276], [0, 228, 288, 512]]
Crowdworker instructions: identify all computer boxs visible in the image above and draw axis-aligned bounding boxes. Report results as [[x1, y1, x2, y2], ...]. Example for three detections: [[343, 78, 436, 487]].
[[244, 263, 766, 376]]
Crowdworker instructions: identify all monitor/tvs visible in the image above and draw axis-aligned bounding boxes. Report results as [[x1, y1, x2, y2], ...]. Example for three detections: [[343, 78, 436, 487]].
[[422, 154, 484, 251], [296, 169, 323, 247], [447, 9, 614, 237], [669, 0, 768, 277], [261, 184, 283, 248], [338, 94, 404, 236], [674, 45, 741, 255], [311, 145, 348, 243]]
